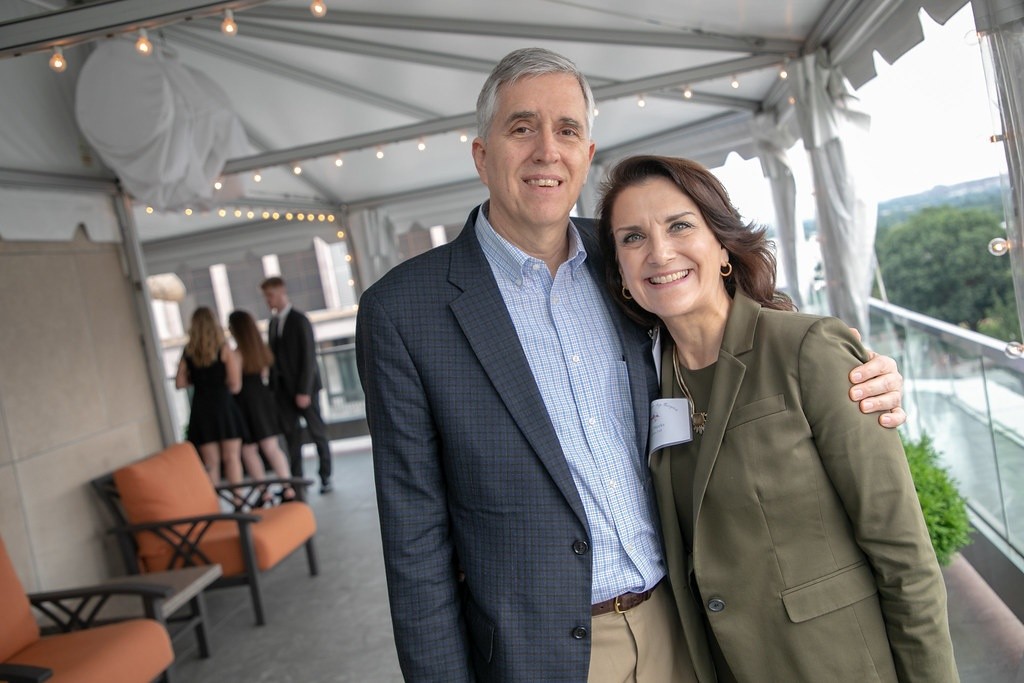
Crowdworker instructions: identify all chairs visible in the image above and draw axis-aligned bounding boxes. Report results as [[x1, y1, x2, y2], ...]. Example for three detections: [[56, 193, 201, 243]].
[[1, 536, 174, 683], [91, 442, 319, 626]]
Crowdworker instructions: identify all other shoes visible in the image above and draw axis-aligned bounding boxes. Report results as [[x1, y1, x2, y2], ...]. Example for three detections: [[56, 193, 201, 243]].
[[320, 482, 333, 493]]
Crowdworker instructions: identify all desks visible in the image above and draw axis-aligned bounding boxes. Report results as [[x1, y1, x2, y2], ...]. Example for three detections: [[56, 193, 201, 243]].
[[39, 563, 222, 659]]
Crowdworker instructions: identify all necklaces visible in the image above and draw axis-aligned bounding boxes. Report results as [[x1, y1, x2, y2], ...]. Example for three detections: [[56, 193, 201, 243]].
[[671, 345, 707, 435]]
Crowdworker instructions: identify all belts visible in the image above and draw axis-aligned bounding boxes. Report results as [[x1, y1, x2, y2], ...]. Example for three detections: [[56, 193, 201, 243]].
[[592, 573, 667, 617]]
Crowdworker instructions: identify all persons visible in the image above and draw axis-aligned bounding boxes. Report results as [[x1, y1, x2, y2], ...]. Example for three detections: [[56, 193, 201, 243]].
[[176, 306, 249, 512], [227, 311, 297, 507], [354, 48, 909, 683], [261, 277, 335, 494], [597, 156, 960, 683]]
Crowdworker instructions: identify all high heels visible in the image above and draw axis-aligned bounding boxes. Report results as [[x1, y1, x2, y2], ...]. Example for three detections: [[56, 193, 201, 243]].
[[280, 487, 295, 504], [253, 493, 274, 510]]
